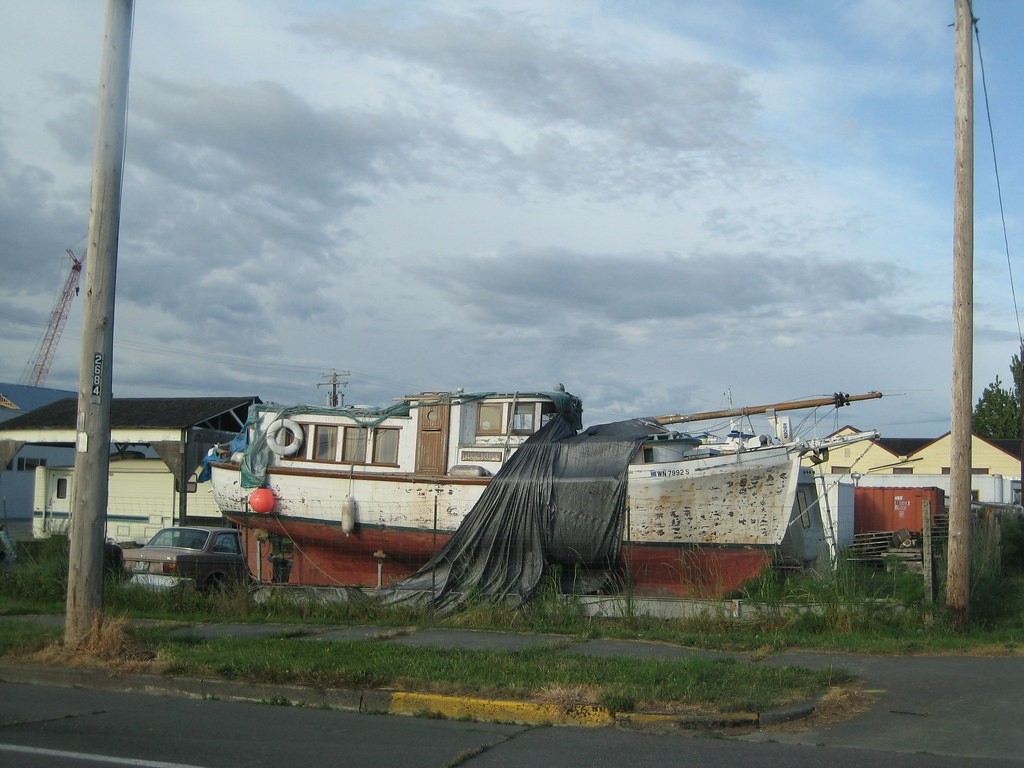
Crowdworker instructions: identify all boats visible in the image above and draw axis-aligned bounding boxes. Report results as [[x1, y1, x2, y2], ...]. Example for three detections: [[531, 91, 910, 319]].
[[207, 383, 945, 618]]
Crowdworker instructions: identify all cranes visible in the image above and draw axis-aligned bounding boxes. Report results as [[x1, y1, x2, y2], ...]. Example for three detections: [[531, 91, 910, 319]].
[[18, 236, 87, 387]]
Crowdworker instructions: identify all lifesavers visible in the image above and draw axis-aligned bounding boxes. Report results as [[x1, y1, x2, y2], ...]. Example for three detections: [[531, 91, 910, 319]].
[[265, 419, 304, 456]]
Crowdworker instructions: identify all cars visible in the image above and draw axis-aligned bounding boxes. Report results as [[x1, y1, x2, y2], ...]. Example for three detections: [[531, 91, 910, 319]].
[[122, 527, 292, 597]]
